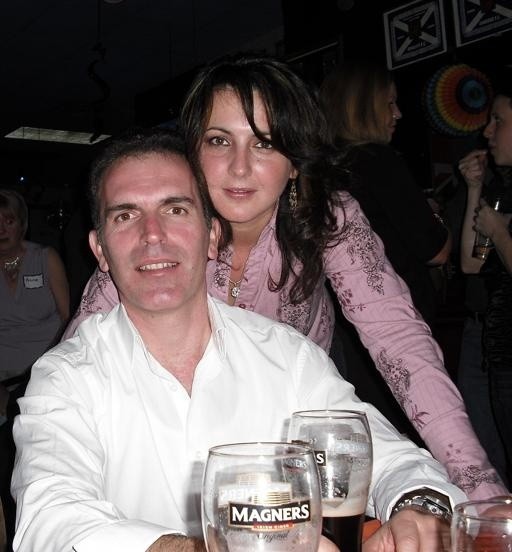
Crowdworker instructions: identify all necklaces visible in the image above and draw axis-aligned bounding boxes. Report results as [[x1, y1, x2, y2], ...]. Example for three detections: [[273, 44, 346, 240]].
[[3, 256, 21, 270], [228, 278, 244, 297]]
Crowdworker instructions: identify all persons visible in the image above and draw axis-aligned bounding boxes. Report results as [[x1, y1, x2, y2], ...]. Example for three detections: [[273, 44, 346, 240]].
[[59, 56, 512, 520], [307, 47, 454, 439], [0, 182, 73, 427], [457, 87, 512, 493], [7, 123, 473, 551]]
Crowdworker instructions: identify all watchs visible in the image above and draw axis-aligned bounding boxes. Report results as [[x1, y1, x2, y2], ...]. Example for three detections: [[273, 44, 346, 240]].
[[388, 488, 453, 525]]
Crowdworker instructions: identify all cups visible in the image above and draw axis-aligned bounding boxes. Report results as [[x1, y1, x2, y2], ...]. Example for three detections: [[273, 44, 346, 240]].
[[450, 499, 512, 552], [200, 408, 373, 552]]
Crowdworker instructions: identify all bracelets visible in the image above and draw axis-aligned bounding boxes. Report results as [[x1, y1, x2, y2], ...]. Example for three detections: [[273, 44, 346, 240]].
[[433, 213, 445, 225]]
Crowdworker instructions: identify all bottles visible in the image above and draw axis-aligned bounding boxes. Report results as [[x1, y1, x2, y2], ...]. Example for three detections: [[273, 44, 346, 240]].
[[473, 198, 502, 259]]
